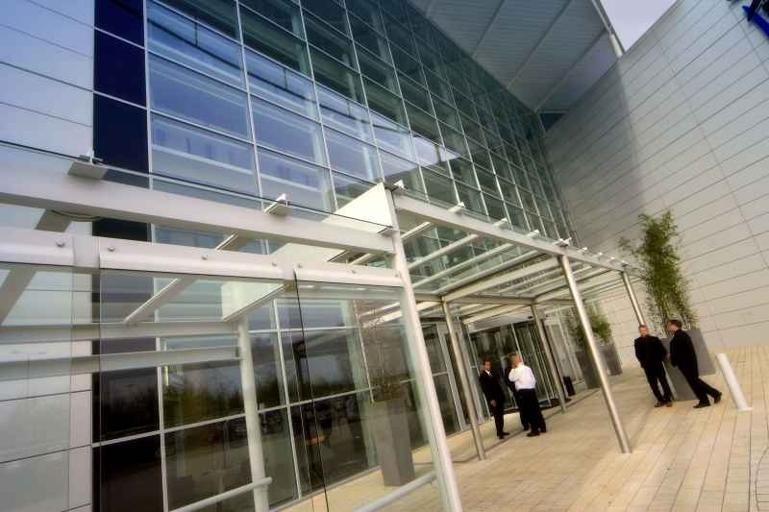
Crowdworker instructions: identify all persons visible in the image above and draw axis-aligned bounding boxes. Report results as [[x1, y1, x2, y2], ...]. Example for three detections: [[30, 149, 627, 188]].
[[633, 325, 673, 408], [479, 358, 510, 439], [508, 355, 547, 436], [503, 353, 539, 431], [666, 319, 722, 409]]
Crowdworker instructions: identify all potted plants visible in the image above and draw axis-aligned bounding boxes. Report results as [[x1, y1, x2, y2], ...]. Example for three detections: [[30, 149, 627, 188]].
[[348, 303, 415, 485], [574, 323, 601, 389], [617, 208, 716, 400], [593, 320, 622, 375]]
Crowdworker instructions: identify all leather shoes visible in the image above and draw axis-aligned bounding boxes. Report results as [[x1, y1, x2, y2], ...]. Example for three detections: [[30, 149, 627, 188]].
[[497, 431, 509, 439], [713, 392, 722, 403], [522, 424, 546, 437], [693, 401, 711, 408], [654, 400, 672, 407]]
